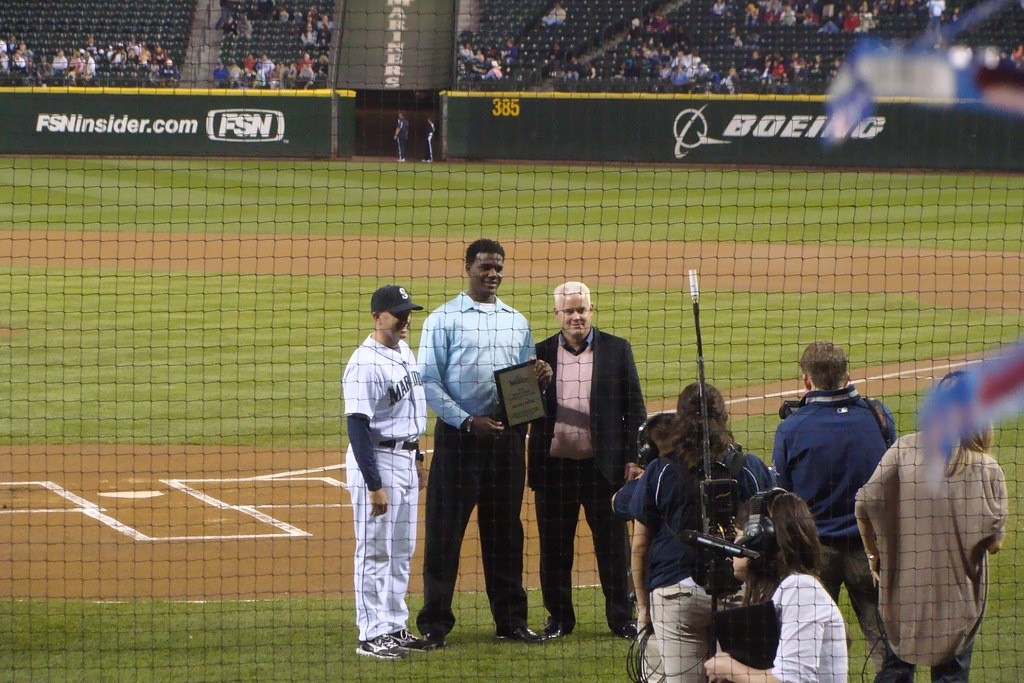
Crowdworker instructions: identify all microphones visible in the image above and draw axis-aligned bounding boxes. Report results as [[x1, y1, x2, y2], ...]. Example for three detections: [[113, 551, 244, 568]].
[[680, 529, 761, 559]]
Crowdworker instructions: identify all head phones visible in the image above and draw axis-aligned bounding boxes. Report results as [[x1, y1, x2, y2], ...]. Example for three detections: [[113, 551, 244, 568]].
[[743, 488, 789, 549], [635, 412, 673, 470]]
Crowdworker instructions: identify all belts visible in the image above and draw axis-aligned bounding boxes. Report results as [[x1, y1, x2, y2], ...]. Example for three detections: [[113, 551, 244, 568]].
[[381, 439, 418, 452]]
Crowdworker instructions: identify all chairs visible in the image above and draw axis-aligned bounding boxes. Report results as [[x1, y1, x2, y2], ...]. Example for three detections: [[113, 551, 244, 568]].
[[454, 0, 1023, 93], [0, 1, 340, 88]]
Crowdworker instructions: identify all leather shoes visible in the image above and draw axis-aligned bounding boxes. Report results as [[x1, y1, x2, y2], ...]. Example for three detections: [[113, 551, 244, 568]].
[[422, 630, 445, 649], [543, 623, 566, 639], [615, 624, 636, 638], [497, 626, 541, 644]]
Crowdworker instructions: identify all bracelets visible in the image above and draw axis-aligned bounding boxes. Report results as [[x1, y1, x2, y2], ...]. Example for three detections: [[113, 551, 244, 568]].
[[466, 416, 474, 432], [395, 134, 398, 136], [867, 552, 880, 562]]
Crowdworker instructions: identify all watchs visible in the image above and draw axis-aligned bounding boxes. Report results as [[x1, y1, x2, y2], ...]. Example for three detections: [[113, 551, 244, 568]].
[[416, 454, 424, 462]]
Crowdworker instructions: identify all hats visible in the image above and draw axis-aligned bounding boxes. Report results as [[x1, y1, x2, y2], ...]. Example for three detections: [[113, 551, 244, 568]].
[[371, 286, 422, 314]]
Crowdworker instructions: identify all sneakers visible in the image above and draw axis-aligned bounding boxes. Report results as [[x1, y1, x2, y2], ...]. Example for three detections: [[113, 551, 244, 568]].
[[387, 630, 424, 653], [355, 635, 409, 659]]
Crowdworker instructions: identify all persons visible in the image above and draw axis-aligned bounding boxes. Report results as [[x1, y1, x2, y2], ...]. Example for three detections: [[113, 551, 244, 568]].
[[703, 487, 848, 683], [854, 370, 1007, 683], [0, 0, 335, 89], [422, 117, 435, 163], [410, 239, 554, 650], [342, 286, 430, 659], [612, 382, 776, 683], [393, 111, 409, 163], [455, 0, 1024, 104], [770, 341, 896, 675], [525, 281, 647, 641]]
